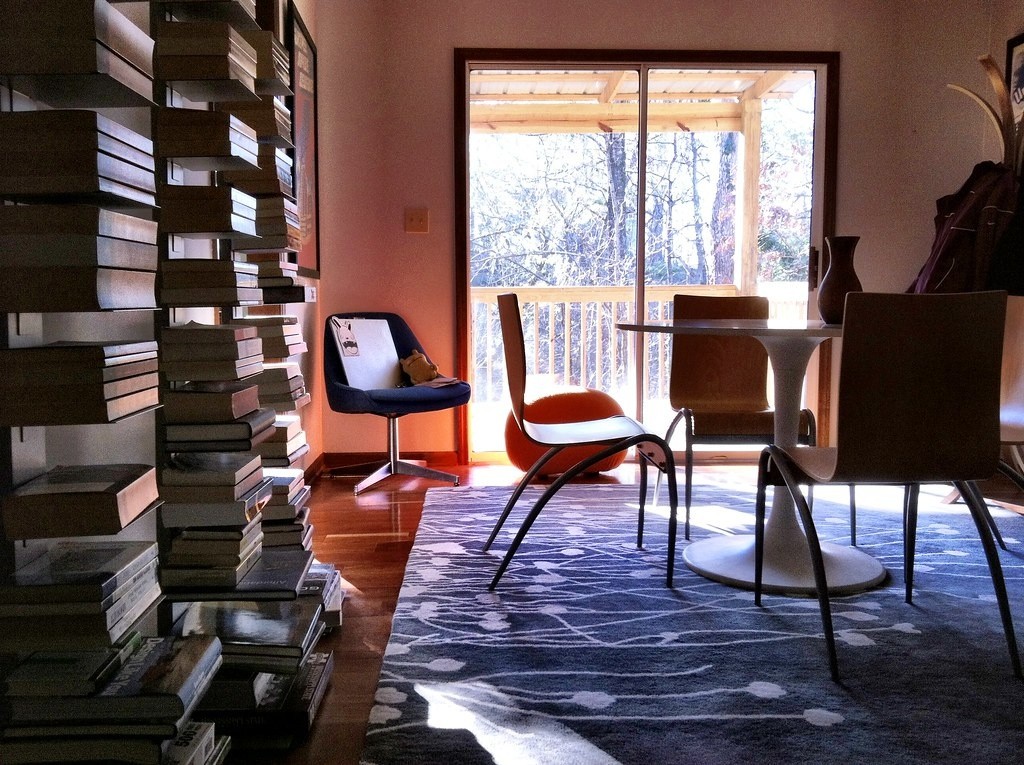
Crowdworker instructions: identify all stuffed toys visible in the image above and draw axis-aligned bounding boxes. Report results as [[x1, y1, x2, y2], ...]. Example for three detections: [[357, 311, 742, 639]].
[[400, 350, 439, 385]]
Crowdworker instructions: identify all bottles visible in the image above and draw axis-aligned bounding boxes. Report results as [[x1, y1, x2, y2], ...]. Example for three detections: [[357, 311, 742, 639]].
[[816, 237, 863, 326]]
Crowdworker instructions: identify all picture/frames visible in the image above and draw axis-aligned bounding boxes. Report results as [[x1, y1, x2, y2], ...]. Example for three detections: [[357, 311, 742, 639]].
[[283, 0, 321, 281], [1004, 31, 1024, 124]]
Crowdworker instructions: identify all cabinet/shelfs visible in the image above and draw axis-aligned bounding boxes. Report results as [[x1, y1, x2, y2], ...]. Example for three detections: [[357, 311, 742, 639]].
[[0, 0, 348, 765]]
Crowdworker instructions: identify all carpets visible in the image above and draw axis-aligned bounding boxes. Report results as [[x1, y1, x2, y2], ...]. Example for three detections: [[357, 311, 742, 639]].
[[359, 478, 1024, 765]]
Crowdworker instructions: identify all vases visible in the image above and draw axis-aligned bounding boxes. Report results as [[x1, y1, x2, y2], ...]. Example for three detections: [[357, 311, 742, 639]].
[[816, 235, 863, 329]]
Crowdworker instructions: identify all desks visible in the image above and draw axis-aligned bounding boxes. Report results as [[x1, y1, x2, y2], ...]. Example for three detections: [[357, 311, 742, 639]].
[[614, 320, 841, 581]]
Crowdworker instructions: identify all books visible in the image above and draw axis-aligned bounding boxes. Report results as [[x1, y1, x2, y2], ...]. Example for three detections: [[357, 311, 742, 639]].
[[0, 0, 358, 765]]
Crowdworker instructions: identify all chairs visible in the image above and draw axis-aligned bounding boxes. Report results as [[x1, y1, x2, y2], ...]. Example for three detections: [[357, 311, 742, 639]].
[[940, 294, 1024, 516], [755, 290, 1024, 683], [482, 292, 678, 595], [651, 292, 818, 541], [318, 311, 471, 496]]
